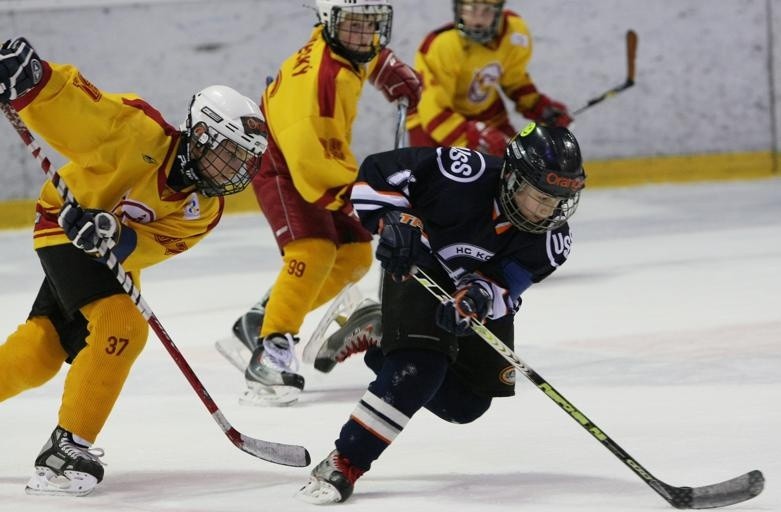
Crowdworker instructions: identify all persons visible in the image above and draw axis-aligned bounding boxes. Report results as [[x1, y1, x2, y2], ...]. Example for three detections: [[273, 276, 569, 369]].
[[404, 0, 572, 159], [215, 0, 423, 409], [299, 122, 586, 505], [0, 36, 269, 498]]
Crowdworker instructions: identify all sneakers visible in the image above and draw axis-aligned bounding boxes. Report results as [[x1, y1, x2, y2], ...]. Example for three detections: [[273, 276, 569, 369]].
[[313, 450, 354, 500], [233, 303, 262, 352], [315, 305, 383, 372], [35, 425, 104, 479], [245, 332, 304, 389]]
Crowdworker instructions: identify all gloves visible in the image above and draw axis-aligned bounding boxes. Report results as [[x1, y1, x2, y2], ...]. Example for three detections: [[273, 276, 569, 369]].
[[58, 202, 120, 255], [524, 96, 568, 127], [467, 120, 505, 155], [0, 38, 43, 101], [369, 49, 422, 112], [437, 279, 494, 336], [376, 210, 431, 282]]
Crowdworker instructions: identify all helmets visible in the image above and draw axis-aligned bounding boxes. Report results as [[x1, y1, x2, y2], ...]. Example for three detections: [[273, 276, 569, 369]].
[[318, 1, 392, 62], [454, 0, 504, 38], [179, 85, 268, 195], [505, 124, 585, 232]]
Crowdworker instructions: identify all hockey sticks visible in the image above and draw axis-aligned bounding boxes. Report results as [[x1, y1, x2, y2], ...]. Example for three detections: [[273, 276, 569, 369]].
[[408, 266, 765, 508], [573, 31, 637, 121], [2, 101, 311, 467]]
